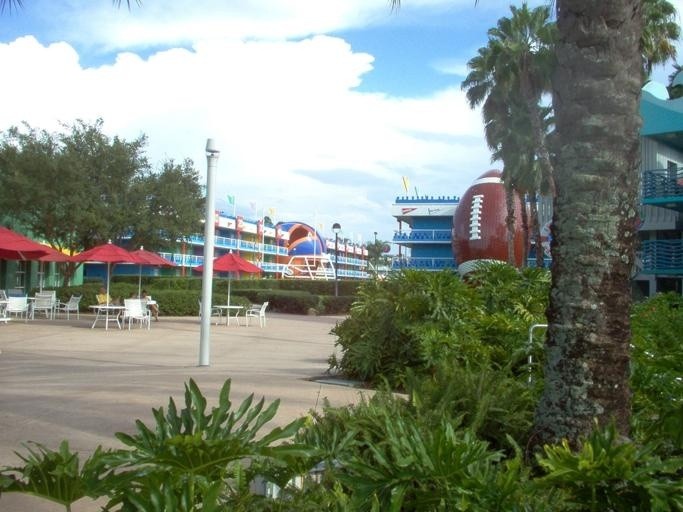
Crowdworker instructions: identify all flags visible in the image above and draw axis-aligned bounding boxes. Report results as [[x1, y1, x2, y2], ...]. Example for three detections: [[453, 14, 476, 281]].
[[227, 195, 234, 204], [270, 207, 275, 215], [250, 201, 256, 209]]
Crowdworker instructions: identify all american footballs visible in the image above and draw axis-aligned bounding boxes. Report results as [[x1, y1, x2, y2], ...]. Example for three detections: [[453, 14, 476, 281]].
[[452, 169, 530, 289]]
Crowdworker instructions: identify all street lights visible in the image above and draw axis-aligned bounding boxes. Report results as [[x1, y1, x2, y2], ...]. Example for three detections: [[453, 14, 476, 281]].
[[332, 222, 341, 296], [198, 135, 221, 368], [373, 231, 377, 245]]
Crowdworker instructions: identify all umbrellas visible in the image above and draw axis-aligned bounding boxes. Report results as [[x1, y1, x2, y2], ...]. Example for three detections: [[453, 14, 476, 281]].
[[0, 226, 50, 261], [129, 245, 177, 298], [66, 239, 151, 306], [37, 246, 69, 293], [192, 249, 264, 306]]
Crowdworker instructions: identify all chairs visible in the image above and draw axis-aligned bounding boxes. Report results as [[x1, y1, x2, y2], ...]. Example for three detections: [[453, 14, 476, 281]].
[[0, 283, 158, 331], [197, 300, 222, 325], [245, 301, 269, 327]]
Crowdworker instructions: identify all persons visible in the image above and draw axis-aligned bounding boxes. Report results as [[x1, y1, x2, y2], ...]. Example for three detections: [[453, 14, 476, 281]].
[[99, 288, 160, 321]]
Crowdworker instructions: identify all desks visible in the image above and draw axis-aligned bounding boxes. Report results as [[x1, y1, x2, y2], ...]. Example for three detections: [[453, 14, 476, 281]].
[[212, 304, 243, 325]]
